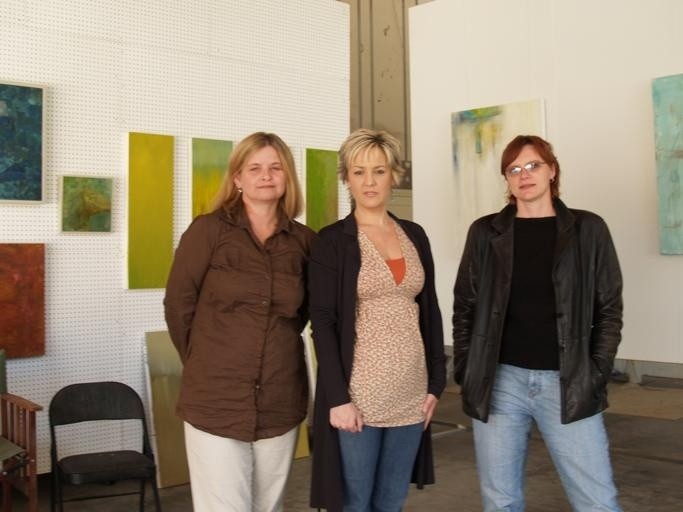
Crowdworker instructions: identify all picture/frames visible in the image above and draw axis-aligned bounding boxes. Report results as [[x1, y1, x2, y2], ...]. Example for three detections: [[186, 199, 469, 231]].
[[0, 81, 46, 205], [57, 177, 115, 235]]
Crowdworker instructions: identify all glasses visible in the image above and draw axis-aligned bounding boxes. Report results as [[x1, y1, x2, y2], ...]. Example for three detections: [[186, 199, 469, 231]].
[[509, 161, 547, 176]]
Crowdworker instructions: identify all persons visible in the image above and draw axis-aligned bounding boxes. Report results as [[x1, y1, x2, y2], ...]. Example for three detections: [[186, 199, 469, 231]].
[[305, 127, 448, 512], [452, 135, 625, 511], [161, 130, 316, 512]]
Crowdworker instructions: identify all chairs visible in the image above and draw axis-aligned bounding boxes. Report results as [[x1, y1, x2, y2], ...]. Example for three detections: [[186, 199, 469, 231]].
[[49, 382, 161, 511]]
